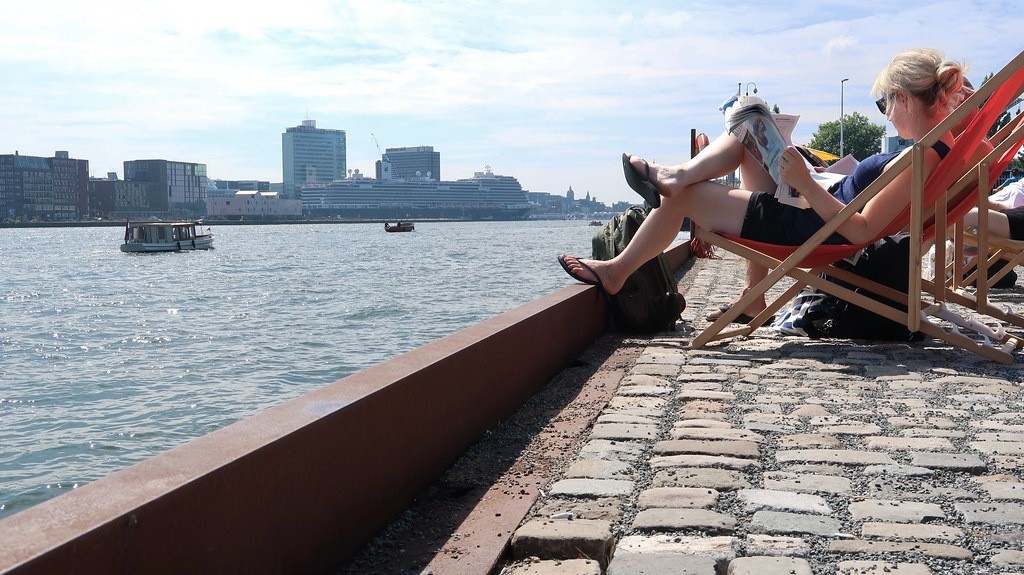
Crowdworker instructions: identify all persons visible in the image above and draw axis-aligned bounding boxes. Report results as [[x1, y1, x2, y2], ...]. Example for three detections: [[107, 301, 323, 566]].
[[557, 46, 970, 297], [933, 168, 1024, 240], [689, 76, 995, 322]]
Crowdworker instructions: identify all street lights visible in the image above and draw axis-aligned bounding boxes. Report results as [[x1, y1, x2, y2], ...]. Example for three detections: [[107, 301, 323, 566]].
[[840, 78, 850, 162]]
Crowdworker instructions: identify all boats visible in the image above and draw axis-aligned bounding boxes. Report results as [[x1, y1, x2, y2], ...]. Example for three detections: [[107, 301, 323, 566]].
[[588, 220, 604, 226], [119, 221, 214, 252], [384, 220, 416, 233]]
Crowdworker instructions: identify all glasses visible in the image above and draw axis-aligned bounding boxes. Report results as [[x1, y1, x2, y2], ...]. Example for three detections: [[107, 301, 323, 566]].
[[874, 91, 895, 114]]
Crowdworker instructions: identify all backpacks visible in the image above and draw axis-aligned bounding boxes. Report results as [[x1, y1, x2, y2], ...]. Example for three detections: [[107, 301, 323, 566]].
[[592, 205, 685, 333]]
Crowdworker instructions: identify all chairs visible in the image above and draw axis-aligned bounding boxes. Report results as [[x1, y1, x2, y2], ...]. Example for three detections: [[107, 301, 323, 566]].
[[694, 48, 1024, 364]]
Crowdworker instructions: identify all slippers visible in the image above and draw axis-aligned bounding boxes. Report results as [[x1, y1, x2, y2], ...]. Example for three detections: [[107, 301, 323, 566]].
[[703, 305, 773, 327], [689, 129, 709, 169], [555, 254, 616, 299], [621, 152, 662, 209]]
